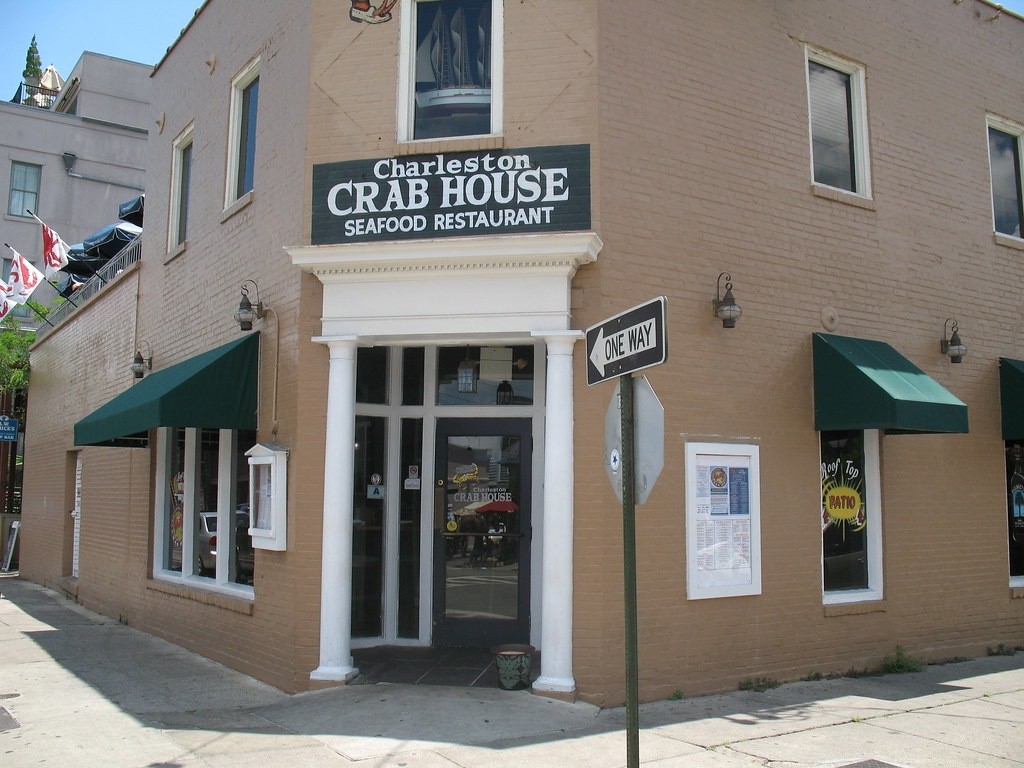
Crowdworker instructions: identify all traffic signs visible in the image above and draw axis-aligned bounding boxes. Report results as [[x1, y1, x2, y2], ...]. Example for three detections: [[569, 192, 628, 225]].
[[584, 295, 667, 387]]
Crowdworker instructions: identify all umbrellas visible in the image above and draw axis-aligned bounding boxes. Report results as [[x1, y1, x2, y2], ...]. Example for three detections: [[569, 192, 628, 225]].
[[54, 274, 89, 300], [119, 193, 145, 230], [82, 222, 143, 259], [58, 240, 102, 276], [454, 500, 519, 531]]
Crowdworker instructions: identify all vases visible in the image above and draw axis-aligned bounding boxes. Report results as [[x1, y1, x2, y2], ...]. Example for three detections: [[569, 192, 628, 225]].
[[491, 644, 536, 691]]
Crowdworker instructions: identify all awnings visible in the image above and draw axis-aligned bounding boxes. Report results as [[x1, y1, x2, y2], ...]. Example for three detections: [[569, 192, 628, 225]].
[[1000, 358, 1024, 440], [72, 331, 261, 447], [811, 333, 973, 433]]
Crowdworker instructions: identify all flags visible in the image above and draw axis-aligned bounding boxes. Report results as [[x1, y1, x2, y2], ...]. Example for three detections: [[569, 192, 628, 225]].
[[0, 276, 18, 324], [6, 251, 46, 306], [40, 224, 70, 280]]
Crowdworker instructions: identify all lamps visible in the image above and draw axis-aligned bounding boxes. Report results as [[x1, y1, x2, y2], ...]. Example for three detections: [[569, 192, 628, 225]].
[[497, 379, 516, 405], [455, 344, 478, 393], [940, 318, 968, 364], [232, 279, 260, 334], [711, 273, 742, 329], [513, 357, 528, 369], [130, 340, 153, 378]]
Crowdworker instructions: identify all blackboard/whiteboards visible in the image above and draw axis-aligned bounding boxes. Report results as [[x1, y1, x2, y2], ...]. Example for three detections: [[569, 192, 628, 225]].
[[1, 522, 18, 571]]
